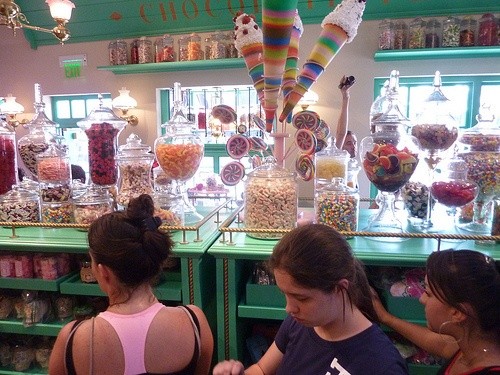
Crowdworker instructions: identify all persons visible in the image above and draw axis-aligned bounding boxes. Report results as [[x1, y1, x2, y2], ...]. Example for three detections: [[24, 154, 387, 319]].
[[213, 224, 410, 375], [49, 194, 214, 375], [369, 248, 500, 375], [336, 75, 361, 188]]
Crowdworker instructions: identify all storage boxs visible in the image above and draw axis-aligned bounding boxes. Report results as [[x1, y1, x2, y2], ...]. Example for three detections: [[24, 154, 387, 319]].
[[385, 288, 427, 321], [407, 362, 442, 375], [244, 274, 287, 308]]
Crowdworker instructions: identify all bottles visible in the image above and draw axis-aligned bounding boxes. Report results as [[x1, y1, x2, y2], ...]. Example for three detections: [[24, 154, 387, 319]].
[[442, 16, 461, 48], [0, 82, 206, 236], [376, 19, 393, 50], [313, 67, 500, 245], [477, 13, 500, 46], [108, 29, 244, 65], [0, 249, 110, 371], [151, 257, 180, 308], [460, 15, 478, 47], [425, 20, 443, 48], [242, 155, 300, 241], [406, 17, 426, 49], [394, 20, 408, 50]]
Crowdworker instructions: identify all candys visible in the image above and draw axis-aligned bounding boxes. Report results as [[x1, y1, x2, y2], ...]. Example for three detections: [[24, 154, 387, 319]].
[[108, 40, 239, 67], [0, 121, 204, 232], [244, 179, 298, 239], [378, 18, 500, 51], [213, 106, 330, 186], [0, 250, 98, 372], [315, 124, 500, 241]]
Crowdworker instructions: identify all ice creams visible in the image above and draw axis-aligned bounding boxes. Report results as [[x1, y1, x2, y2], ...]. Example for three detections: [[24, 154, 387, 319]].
[[232, 0, 367, 133]]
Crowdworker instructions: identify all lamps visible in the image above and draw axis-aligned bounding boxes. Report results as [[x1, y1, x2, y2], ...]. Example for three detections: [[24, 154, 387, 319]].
[[111, 86, 138, 128], [0, 97, 30, 131], [296, 88, 319, 111], [0, 0, 76, 48]]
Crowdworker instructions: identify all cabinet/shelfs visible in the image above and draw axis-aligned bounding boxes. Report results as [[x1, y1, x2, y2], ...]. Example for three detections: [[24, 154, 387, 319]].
[[205, 196, 500, 375], [0, 194, 239, 375]]
